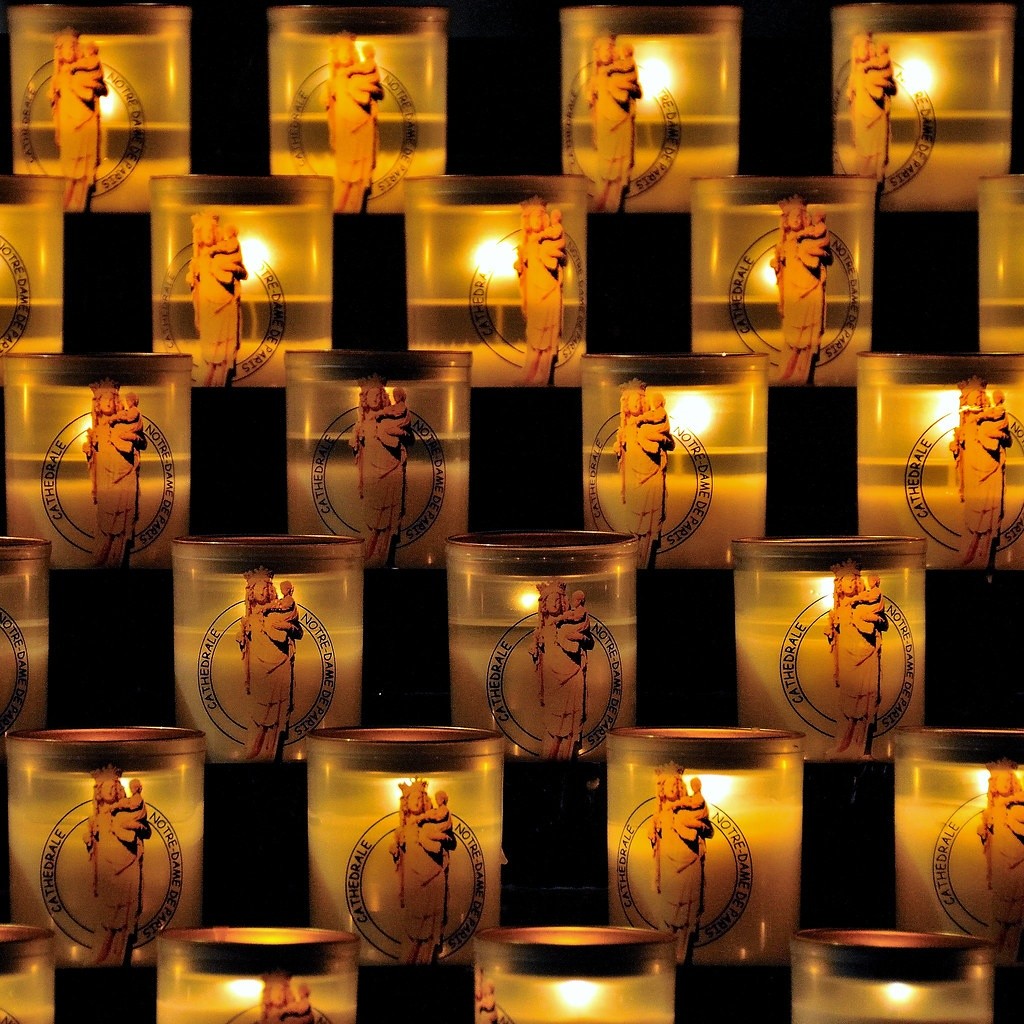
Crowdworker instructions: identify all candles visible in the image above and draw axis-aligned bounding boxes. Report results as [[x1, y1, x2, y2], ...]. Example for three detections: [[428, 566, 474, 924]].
[[1, 1, 1024, 1023]]
